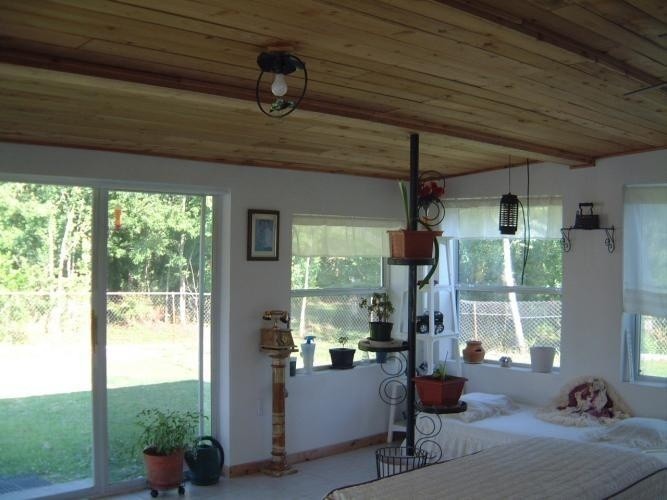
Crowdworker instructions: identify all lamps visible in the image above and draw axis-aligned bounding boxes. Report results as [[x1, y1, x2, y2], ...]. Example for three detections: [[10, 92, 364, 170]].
[[499, 153, 520, 236], [255, 52, 308, 118]]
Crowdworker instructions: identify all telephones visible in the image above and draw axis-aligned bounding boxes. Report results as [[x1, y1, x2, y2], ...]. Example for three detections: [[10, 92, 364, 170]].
[[260, 310, 297, 348]]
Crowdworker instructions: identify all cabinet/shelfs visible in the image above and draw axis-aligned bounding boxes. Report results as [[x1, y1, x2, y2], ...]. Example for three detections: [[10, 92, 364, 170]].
[[357, 132, 467, 445]]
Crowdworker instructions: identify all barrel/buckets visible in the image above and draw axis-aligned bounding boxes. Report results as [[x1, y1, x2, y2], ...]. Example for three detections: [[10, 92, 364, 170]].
[[143, 444, 185, 489], [530, 345, 556, 374]]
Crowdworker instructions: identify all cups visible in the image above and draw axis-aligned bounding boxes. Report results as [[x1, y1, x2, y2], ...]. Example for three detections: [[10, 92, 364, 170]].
[[290, 356, 298, 377]]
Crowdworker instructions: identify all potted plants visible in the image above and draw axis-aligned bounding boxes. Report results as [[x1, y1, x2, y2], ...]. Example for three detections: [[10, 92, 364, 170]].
[[328, 335, 355, 367], [129, 408, 210, 490], [413, 350, 468, 408], [358, 291, 395, 340]]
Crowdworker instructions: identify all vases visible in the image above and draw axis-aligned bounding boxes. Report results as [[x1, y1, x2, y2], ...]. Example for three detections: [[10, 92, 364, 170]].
[[530, 347, 555, 373], [386, 230, 442, 259], [463, 341, 485, 363]]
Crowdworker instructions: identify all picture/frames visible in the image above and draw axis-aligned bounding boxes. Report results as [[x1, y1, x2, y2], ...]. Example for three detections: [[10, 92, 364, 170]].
[[246, 208, 279, 261]]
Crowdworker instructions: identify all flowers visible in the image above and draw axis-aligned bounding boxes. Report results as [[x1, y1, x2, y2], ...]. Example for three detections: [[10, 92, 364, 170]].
[[387, 171, 445, 290]]
